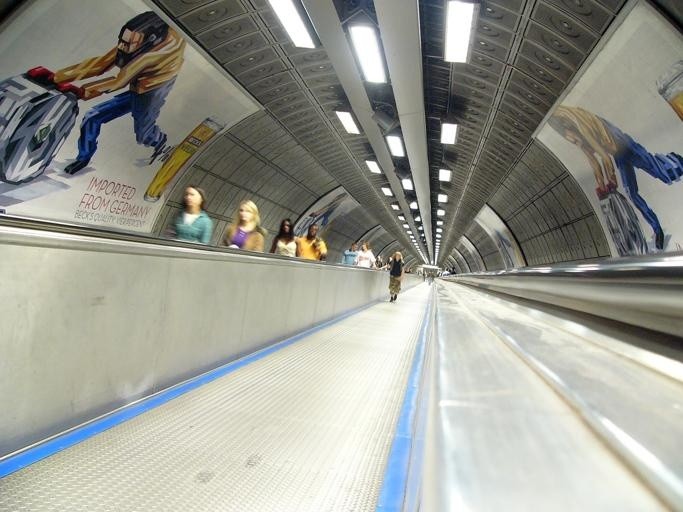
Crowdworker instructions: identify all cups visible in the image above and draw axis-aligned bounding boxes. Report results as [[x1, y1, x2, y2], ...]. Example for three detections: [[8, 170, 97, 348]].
[[655, 58, 682, 121], [141, 110, 226, 204]]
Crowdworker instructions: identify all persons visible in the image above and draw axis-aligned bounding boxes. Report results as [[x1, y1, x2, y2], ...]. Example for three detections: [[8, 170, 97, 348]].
[[354, 240, 377, 271], [379, 251, 405, 302], [22, 11, 188, 174], [542, 104, 683, 254], [164, 187, 213, 246], [292, 222, 328, 260], [270, 220, 303, 259], [375, 254, 457, 285], [341, 242, 359, 266], [221, 199, 265, 252]]
